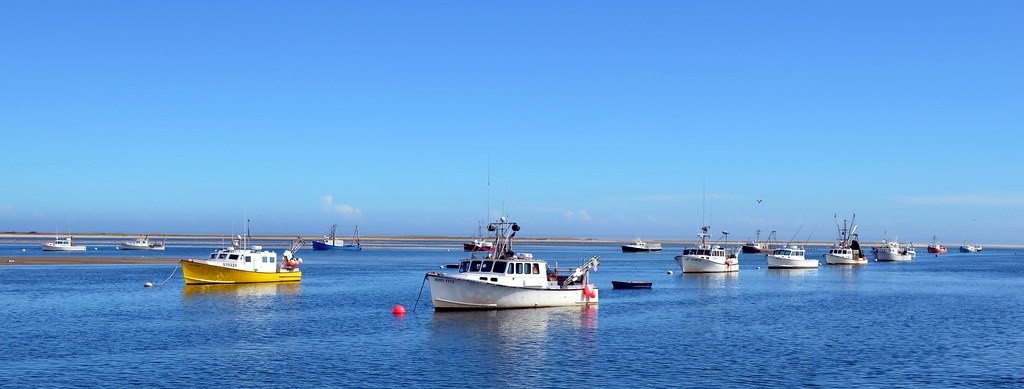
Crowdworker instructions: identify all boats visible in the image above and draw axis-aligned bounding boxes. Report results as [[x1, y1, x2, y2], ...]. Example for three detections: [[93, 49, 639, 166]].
[[620, 236, 663, 252], [959, 240, 984, 253], [116, 234, 165, 251], [762, 222, 819, 269], [871, 230, 917, 261], [176, 219, 307, 286], [742, 229, 781, 254], [425, 215, 602, 312], [674, 188, 743, 274], [312, 224, 363, 251], [42, 221, 87, 251], [824, 212, 870, 265], [927, 236, 949, 255], [610, 278, 653, 290]]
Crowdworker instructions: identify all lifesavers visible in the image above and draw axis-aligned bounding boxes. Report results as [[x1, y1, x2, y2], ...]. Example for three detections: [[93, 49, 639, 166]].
[[727, 260, 733, 266], [549, 274, 557, 281]]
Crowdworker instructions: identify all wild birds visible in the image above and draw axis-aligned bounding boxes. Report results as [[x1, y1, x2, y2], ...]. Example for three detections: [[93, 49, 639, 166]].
[[756, 199, 763, 203]]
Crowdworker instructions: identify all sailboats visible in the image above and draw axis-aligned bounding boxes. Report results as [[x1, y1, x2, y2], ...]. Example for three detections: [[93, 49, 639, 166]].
[[456, 158, 496, 252]]
